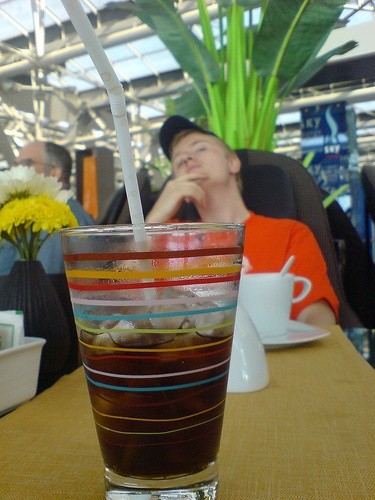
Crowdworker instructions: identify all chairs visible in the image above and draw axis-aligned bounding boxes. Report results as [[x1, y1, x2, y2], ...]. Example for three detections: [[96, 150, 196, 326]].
[[160, 149, 347, 333], [101, 168, 152, 225]]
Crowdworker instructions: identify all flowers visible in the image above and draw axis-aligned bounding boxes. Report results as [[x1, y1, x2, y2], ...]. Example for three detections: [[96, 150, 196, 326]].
[[0, 165, 78, 261]]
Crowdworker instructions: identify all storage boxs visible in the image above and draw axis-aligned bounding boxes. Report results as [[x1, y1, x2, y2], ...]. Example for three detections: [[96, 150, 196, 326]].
[[0, 337, 46, 418]]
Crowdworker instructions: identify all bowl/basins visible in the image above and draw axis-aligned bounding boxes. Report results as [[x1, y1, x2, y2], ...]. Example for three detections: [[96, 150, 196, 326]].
[[225, 303, 272, 393]]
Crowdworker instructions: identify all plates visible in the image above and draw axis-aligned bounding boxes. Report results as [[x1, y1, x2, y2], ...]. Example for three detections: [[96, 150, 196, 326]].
[[262, 319, 331, 350]]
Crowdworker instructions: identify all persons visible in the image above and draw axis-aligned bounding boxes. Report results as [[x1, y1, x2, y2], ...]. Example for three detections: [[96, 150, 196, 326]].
[[116, 114, 340, 328], [0, 141, 108, 276]]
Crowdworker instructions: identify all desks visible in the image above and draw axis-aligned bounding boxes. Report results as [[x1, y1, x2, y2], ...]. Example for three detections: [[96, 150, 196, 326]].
[[0, 324, 375, 500]]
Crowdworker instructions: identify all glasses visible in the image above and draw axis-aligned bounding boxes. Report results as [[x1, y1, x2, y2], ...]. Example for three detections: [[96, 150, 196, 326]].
[[12, 159, 55, 168]]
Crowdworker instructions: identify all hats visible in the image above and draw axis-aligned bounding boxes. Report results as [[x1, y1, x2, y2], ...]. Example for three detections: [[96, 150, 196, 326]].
[[159, 116, 208, 160]]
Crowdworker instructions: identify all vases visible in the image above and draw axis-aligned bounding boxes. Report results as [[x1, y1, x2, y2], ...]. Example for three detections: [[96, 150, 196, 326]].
[[0, 260, 69, 379]]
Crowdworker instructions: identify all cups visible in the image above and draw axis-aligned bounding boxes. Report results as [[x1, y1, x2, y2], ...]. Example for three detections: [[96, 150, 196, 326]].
[[237, 272, 312, 339], [60, 221, 246, 500]]
